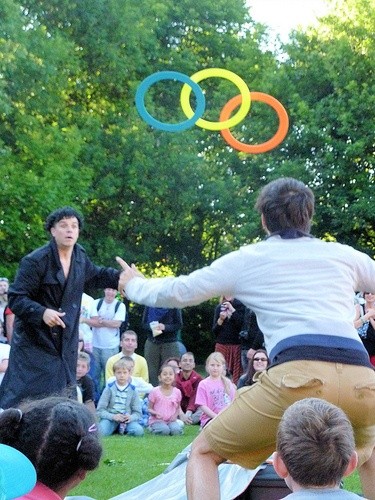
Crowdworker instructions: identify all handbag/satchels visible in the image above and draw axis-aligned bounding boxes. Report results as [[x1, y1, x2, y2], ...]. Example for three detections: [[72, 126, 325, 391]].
[[357, 304, 369, 338]]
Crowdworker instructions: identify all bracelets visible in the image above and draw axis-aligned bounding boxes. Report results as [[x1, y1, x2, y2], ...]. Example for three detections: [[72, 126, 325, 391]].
[[360, 316, 366, 324]]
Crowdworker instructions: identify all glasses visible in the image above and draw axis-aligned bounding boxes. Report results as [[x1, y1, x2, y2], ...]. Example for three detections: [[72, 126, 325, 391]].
[[254, 358, 268, 361], [0, 277, 8, 281]]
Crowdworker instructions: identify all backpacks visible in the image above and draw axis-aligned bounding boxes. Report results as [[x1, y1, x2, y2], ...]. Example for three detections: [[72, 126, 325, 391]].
[[97, 296, 128, 336]]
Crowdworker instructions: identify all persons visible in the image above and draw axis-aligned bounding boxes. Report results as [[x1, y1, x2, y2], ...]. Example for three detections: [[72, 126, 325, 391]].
[[0, 208, 120, 409], [76, 287, 269, 437], [354, 291, 375, 362], [0, 396, 103, 500], [271, 398, 372, 500], [115, 176, 375, 500]]
